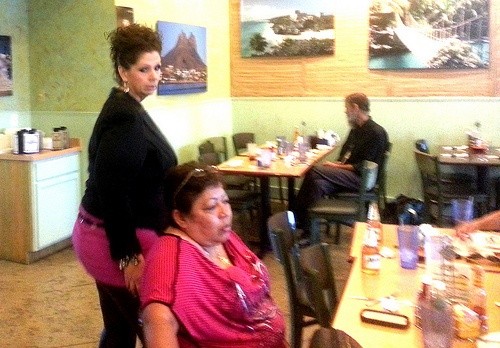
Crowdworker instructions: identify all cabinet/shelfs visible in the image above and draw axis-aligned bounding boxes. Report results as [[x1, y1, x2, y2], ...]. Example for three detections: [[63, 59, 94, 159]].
[[0, 147, 83, 265]]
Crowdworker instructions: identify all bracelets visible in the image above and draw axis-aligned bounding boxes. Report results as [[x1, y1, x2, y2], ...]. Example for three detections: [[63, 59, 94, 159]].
[[119, 254, 140, 269]]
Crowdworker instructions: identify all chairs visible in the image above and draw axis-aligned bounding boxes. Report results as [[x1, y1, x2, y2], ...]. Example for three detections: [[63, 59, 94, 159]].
[[198, 132, 500, 348]]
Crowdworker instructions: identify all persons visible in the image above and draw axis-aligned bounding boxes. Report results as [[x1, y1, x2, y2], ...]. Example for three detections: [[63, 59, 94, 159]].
[[141, 162, 291, 348], [294, 93, 388, 245], [73, 24, 177, 348], [456, 212, 500, 237]]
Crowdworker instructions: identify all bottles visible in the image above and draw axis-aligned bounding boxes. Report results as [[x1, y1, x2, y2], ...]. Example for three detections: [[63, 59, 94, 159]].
[[294, 127, 299, 151], [413, 280, 430, 336], [52, 128, 63, 150], [62, 127, 69, 148], [366, 199, 384, 248], [362, 221, 381, 274], [470, 265, 487, 331]]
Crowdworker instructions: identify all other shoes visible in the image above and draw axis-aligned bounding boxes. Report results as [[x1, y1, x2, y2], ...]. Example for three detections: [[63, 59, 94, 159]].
[[298, 237, 319, 249]]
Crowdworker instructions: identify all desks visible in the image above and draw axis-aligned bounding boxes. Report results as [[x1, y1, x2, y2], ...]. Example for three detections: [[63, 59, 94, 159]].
[[437, 143, 500, 222], [213, 141, 337, 259], [330, 224, 500, 348]]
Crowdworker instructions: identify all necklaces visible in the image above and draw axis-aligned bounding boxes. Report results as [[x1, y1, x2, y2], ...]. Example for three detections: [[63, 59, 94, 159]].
[[205, 246, 228, 263]]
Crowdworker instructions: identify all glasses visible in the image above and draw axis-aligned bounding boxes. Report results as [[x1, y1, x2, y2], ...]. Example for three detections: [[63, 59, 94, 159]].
[[171, 164, 219, 209]]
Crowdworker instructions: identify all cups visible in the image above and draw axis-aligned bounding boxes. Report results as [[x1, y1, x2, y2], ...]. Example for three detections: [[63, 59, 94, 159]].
[[276, 135, 288, 156], [422, 304, 455, 348], [398, 225, 419, 269], [298, 142, 308, 162], [452, 199, 474, 223]]
